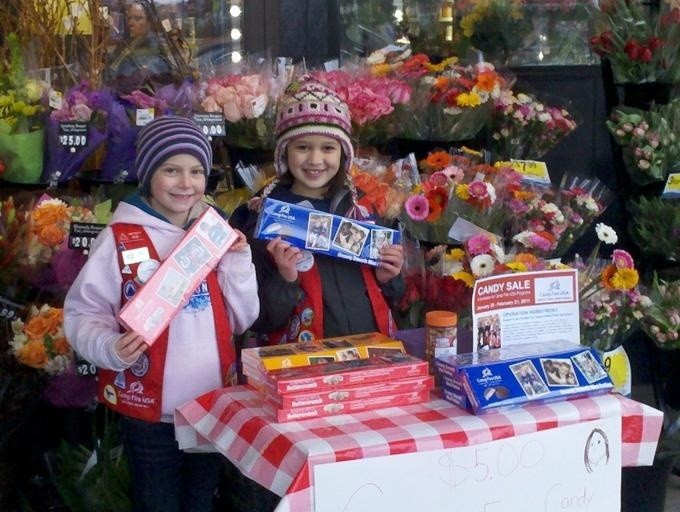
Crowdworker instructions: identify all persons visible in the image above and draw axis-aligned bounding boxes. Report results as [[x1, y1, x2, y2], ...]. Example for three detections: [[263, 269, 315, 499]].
[[478, 325, 501, 358], [235, 77, 407, 345], [199, 222, 228, 247], [61, 113, 265, 510], [310, 220, 365, 254], [102, 0, 188, 105], [521, 356, 575, 395]]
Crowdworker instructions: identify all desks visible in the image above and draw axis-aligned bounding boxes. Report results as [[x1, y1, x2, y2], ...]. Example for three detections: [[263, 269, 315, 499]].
[[216, 385, 622, 512]]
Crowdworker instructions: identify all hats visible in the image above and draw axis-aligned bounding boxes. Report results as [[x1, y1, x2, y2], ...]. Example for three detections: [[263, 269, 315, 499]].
[[135, 113, 213, 192], [271, 73, 355, 178]]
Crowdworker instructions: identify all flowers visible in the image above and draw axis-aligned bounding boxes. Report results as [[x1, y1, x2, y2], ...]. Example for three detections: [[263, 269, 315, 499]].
[[0, 1, 680, 379]]
